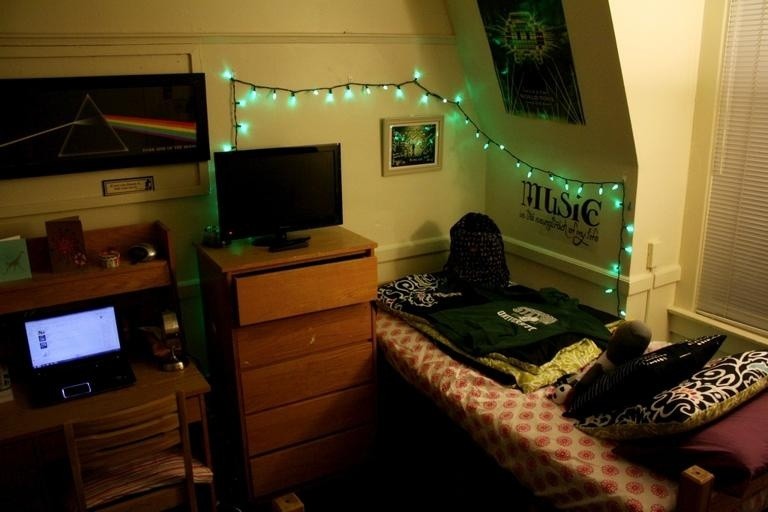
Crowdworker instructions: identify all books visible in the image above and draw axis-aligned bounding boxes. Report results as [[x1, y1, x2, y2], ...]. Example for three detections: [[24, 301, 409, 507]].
[[43, 216, 89, 272], [0, 234, 33, 283]]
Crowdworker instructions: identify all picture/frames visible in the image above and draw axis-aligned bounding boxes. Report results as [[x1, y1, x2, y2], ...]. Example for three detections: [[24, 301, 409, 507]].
[[382, 116, 445, 176]]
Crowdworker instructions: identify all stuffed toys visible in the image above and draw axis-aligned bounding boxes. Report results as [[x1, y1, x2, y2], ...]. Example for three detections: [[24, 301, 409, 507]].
[[550, 320, 653, 411]]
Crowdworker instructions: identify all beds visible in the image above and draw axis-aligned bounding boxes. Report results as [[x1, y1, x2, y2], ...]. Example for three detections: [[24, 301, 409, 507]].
[[375, 268, 768, 512]]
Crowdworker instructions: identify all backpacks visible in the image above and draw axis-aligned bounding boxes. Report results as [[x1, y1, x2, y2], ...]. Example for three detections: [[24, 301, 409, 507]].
[[442, 212, 511, 290]]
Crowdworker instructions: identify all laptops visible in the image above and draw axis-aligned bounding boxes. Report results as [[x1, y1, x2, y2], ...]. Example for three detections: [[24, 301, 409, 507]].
[[18, 302, 137, 410]]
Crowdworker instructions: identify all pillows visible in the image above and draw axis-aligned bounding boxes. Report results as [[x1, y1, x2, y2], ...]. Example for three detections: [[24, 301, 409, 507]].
[[559, 333, 726, 418], [572, 347, 768, 440], [611, 387, 768, 497]]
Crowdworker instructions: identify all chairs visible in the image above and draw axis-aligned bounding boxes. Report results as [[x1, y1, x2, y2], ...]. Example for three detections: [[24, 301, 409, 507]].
[[57, 388, 198, 512]]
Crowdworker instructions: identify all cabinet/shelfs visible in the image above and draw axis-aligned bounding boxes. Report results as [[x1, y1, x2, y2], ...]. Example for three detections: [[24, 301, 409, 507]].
[[0, 220, 218, 512], [190, 226, 383, 507]]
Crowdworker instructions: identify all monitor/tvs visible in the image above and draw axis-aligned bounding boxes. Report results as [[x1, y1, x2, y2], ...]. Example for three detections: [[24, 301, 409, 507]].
[[213, 142, 344, 247]]
